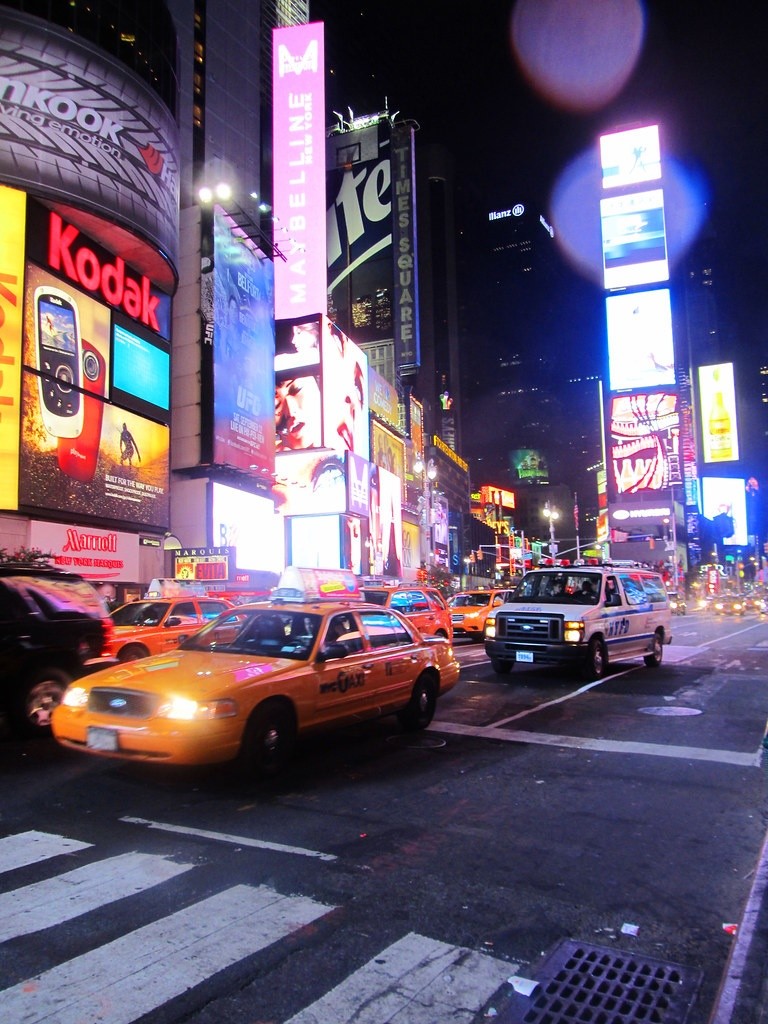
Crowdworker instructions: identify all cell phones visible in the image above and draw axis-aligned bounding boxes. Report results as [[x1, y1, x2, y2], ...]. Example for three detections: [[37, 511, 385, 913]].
[[34, 286, 84, 437], [58, 339, 106, 483]]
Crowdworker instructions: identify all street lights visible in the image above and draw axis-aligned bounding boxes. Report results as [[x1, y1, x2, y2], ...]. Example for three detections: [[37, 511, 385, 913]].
[[412, 450, 439, 588], [542, 499, 560, 567]]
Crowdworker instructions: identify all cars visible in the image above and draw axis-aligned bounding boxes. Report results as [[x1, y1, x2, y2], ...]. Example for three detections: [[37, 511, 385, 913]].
[[109, 596, 251, 665], [52, 597, 461, 782], [668, 587, 768, 617], [446, 588, 515, 641], [358, 586, 454, 650]]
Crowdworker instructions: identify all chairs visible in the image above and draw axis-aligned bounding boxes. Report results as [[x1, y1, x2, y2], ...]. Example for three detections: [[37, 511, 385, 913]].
[[548, 580, 571, 598], [237, 616, 284, 652]]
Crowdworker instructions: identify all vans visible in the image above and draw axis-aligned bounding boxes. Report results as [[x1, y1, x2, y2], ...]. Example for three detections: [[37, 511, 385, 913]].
[[484, 564, 675, 681]]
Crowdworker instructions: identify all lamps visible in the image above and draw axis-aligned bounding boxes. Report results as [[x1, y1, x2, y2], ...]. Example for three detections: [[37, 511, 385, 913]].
[[240, 192, 257, 203], [243, 204, 272, 214], [274, 238, 298, 246], [280, 248, 306, 255], [251, 216, 280, 224], [262, 227, 289, 235]]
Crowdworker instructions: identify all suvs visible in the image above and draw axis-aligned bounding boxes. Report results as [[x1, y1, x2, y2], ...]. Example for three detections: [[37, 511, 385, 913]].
[[0, 564, 114, 732]]
